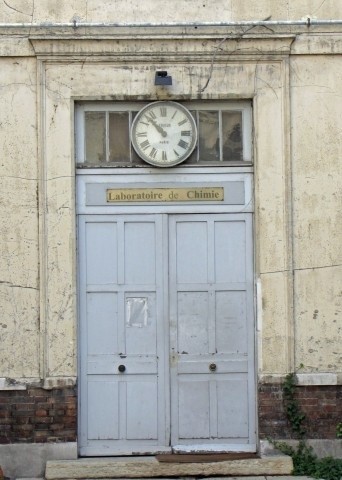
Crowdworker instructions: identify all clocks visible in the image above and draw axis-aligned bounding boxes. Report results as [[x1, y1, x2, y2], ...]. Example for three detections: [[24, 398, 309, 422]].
[[129, 101, 198, 169]]
